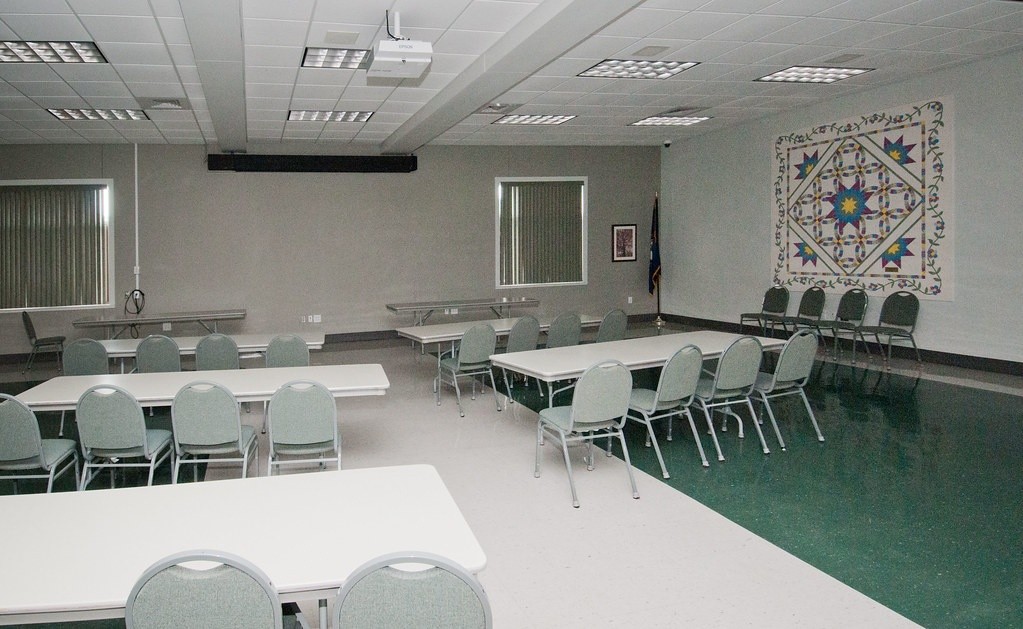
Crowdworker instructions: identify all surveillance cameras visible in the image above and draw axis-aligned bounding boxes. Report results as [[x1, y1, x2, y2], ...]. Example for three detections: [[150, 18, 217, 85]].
[[663, 140, 672, 147]]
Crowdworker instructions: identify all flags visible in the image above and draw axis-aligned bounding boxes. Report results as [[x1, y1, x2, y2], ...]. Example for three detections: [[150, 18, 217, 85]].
[[649, 192, 662, 295]]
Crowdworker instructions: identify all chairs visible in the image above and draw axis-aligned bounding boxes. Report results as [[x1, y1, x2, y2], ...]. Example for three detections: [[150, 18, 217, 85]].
[[0, 285, 923, 629], [21, 311, 66, 374]]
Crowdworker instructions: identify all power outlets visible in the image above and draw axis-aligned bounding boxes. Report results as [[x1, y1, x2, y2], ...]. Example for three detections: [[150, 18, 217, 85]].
[[445, 309, 450, 315], [307, 316, 312, 322], [162, 323, 171, 330], [314, 315, 321, 323], [451, 308, 458, 315], [134, 291, 139, 299]]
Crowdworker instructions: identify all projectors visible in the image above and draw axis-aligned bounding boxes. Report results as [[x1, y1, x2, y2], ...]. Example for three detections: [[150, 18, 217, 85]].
[[365, 40, 433, 78]]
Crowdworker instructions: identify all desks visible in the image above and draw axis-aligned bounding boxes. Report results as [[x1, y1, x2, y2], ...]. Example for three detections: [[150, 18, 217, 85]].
[[5, 363, 390, 432], [91, 332, 325, 374], [489, 329, 789, 444], [72, 309, 245, 341], [385, 297, 539, 354], [394, 313, 604, 393], [0, 466, 487, 628]]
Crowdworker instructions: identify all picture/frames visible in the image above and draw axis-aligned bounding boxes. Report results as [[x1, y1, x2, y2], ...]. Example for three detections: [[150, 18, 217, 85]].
[[611, 224, 637, 262]]
